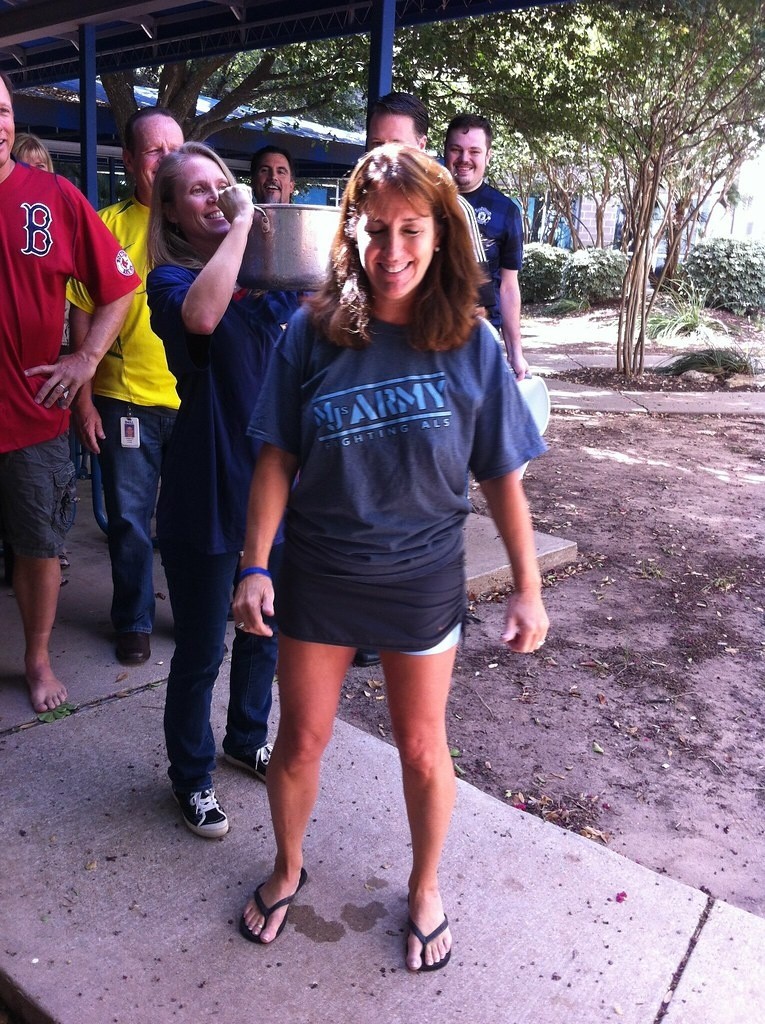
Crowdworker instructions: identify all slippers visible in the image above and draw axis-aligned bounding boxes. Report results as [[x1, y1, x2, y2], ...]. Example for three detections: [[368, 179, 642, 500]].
[[240, 868, 307, 944], [405, 892, 452, 971]]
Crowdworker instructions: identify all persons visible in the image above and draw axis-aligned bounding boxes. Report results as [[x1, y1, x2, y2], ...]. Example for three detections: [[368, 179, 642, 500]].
[[432, 113, 531, 383], [67, 102, 185, 666], [134, 138, 325, 839], [0, 74, 143, 712], [125, 424, 134, 437], [232, 141, 551, 973], [250, 145, 297, 205], [4, 133, 76, 595], [353, 93, 497, 665]]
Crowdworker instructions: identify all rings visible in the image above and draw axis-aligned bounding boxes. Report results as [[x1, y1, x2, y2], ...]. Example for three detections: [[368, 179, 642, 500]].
[[537, 640, 545, 645], [59, 384, 70, 399], [235, 622, 245, 628]]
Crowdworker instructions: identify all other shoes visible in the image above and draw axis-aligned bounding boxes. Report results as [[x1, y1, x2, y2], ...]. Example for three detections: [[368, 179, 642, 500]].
[[59, 553, 70, 569], [360, 650, 380, 666], [60, 576, 69, 587]]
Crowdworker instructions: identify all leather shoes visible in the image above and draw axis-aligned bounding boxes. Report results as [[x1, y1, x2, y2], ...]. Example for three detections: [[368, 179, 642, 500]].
[[117, 631, 151, 663]]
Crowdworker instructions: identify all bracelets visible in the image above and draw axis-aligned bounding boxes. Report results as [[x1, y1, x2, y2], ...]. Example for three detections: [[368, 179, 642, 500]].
[[239, 567, 273, 580]]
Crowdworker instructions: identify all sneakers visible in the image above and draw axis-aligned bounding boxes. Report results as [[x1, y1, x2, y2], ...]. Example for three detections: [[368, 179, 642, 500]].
[[223, 744, 273, 782], [170, 781, 229, 837]]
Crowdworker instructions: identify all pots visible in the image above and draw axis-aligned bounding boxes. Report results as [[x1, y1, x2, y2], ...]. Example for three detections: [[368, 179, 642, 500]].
[[238, 190, 352, 288]]
